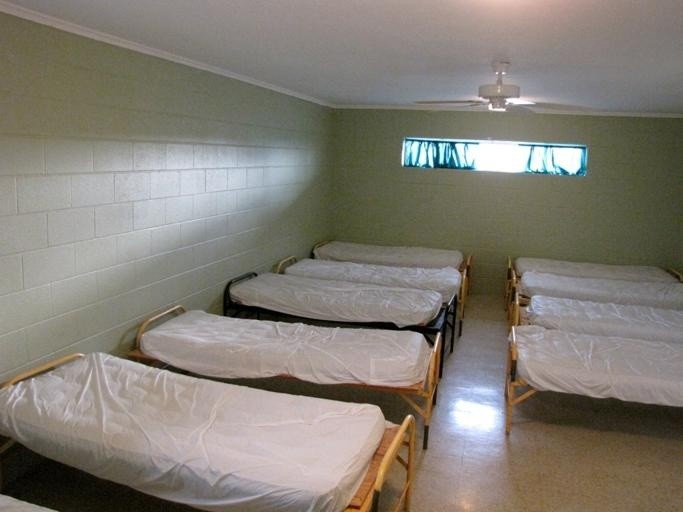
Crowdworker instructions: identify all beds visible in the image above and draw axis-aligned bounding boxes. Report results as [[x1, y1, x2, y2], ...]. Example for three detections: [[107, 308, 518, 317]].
[[223, 272, 458, 378], [504, 255, 682, 434], [1, 352, 417, 512], [278, 256, 466, 352], [128, 306, 442, 451], [310, 239, 472, 336]]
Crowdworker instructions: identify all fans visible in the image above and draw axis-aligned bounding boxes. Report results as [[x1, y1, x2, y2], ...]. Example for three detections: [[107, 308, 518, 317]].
[[413, 62, 593, 115]]
